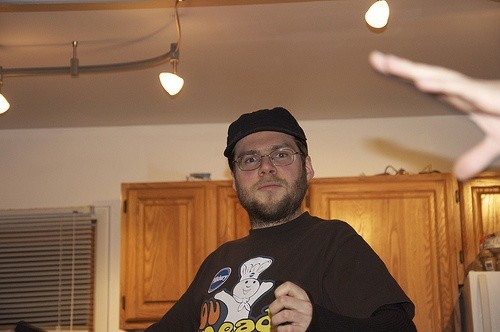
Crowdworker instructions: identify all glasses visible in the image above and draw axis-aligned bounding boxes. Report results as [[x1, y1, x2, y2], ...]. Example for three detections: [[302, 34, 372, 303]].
[[232, 148, 305, 171]]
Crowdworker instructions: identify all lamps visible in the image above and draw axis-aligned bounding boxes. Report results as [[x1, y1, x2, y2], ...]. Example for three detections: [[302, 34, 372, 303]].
[[365, 0, 390, 29], [0, 83, 10, 115], [159, 60, 184, 96]]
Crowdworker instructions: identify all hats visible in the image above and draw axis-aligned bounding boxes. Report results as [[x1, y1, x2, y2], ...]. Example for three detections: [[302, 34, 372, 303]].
[[224, 107, 306, 156]]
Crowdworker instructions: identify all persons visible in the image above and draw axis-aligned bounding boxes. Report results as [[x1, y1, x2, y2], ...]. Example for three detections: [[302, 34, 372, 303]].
[[370, 51, 500, 181], [143, 107, 418, 332]]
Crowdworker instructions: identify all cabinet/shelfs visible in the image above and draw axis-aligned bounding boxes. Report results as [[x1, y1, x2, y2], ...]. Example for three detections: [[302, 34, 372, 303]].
[[121, 168, 500, 332]]
